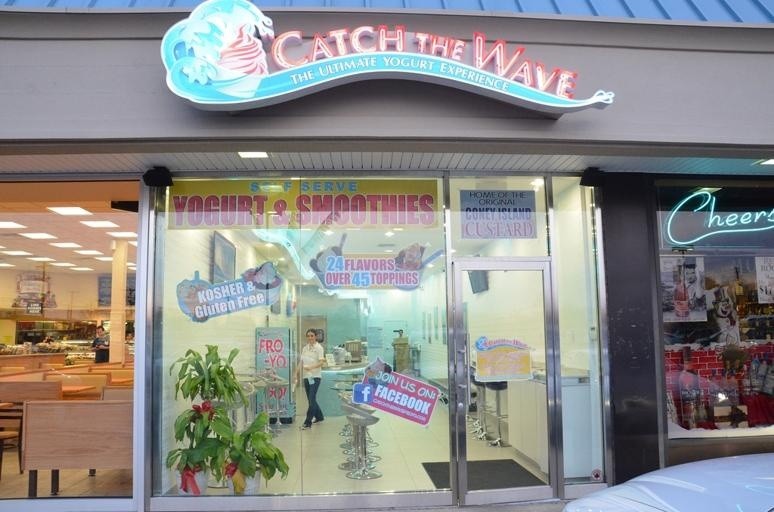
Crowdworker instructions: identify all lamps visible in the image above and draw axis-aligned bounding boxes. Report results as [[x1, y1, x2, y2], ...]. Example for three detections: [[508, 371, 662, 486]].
[[143, 165, 173, 188], [580, 166, 604, 187]]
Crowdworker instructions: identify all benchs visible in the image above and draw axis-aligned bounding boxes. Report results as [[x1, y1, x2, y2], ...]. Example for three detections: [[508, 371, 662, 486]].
[[0, 364, 134, 498]]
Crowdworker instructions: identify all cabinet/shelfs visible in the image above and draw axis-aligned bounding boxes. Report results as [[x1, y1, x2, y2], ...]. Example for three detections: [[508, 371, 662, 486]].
[[507, 367, 591, 484]]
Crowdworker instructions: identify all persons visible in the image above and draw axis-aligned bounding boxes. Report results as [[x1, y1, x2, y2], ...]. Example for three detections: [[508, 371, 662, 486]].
[[90, 325, 109, 362], [681, 256, 706, 313], [293, 329, 324, 430], [124, 330, 134, 344]]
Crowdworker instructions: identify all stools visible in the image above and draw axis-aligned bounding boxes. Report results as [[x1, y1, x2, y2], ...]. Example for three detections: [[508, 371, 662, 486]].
[[469, 373, 508, 448]]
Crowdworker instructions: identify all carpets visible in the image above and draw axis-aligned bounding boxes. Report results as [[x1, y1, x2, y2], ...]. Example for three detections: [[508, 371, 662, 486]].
[[422, 459, 546, 489]]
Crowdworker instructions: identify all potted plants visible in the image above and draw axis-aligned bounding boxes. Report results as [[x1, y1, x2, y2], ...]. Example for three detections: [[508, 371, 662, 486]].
[[165, 344, 290, 496]]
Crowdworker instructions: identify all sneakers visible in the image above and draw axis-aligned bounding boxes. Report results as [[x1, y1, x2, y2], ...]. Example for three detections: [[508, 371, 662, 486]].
[[300, 423, 311, 430], [313, 415, 324, 423]]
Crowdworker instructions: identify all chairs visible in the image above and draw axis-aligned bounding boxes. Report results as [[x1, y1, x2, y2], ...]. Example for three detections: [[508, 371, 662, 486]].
[[337, 392, 382, 481]]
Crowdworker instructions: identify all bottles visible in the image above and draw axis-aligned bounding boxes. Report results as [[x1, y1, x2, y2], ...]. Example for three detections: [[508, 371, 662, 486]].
[[709, 351, 774, 406], [674, 263, 690, 318], [678, 346, 701, 429]]
